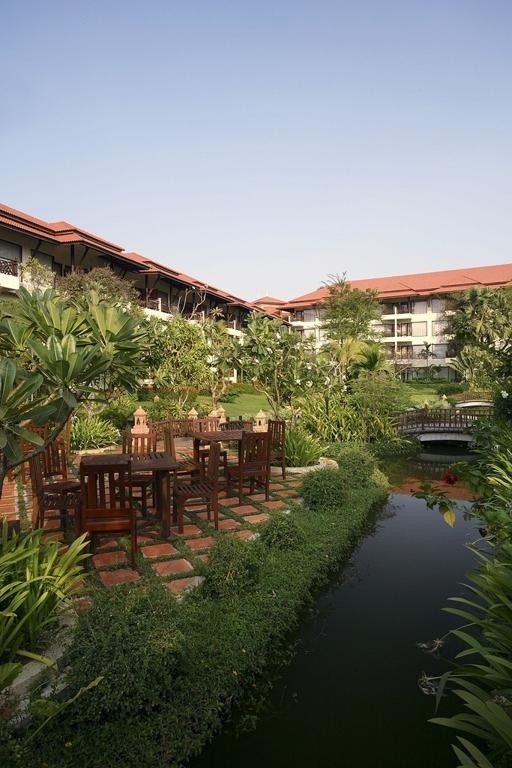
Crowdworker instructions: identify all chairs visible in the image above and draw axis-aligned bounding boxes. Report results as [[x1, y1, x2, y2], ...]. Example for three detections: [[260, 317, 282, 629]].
[[28, 418, 285, 570]]
